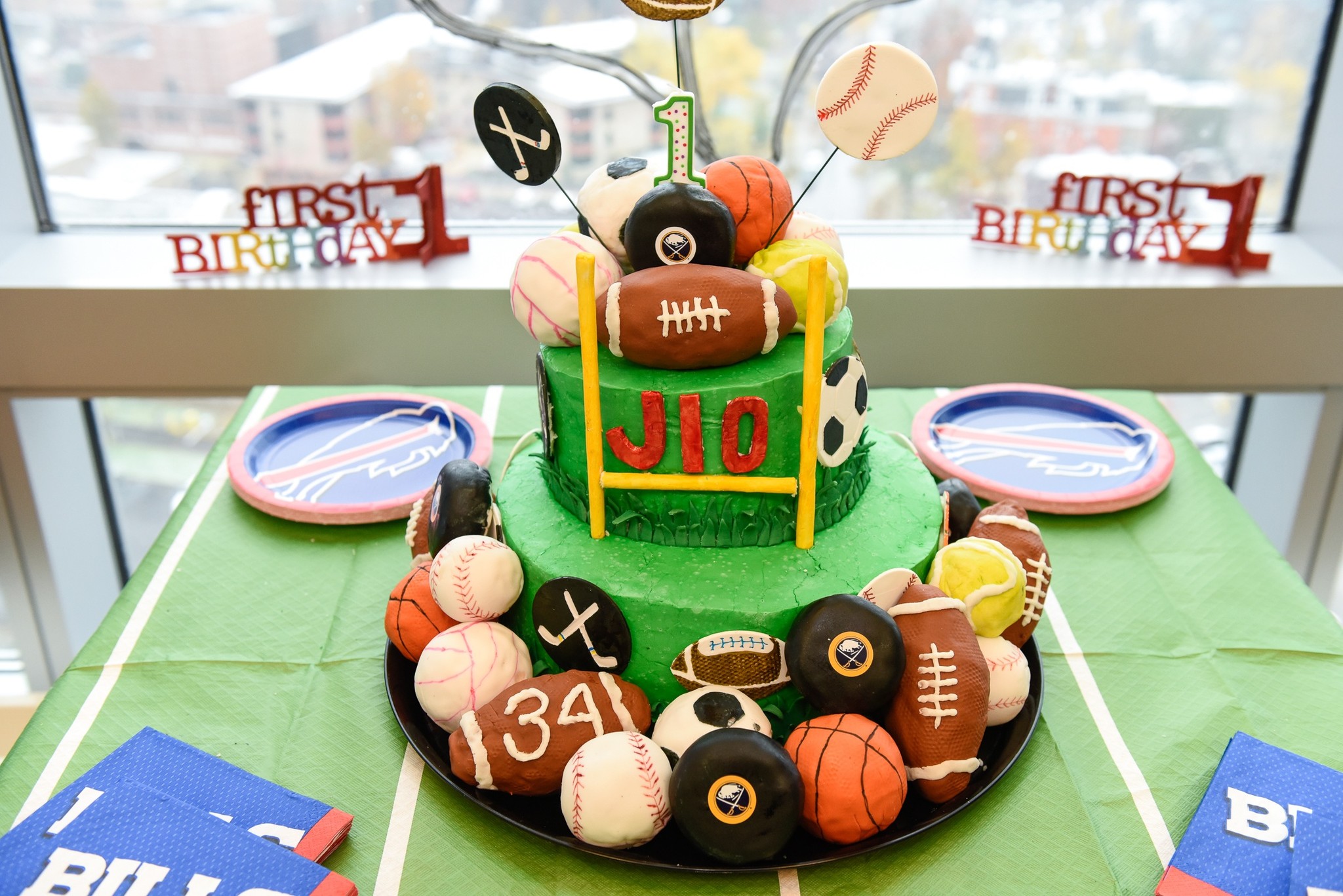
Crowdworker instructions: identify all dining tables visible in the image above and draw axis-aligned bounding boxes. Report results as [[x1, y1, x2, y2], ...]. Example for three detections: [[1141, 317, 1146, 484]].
[[3, 384, 1340, 893]]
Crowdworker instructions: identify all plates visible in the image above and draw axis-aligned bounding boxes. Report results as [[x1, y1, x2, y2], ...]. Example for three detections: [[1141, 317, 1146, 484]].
[[381, 571, 1048, 878]]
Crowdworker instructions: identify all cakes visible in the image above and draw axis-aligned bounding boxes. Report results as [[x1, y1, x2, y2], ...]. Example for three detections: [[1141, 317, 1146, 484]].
[[383, 0, 1054, 864]]
